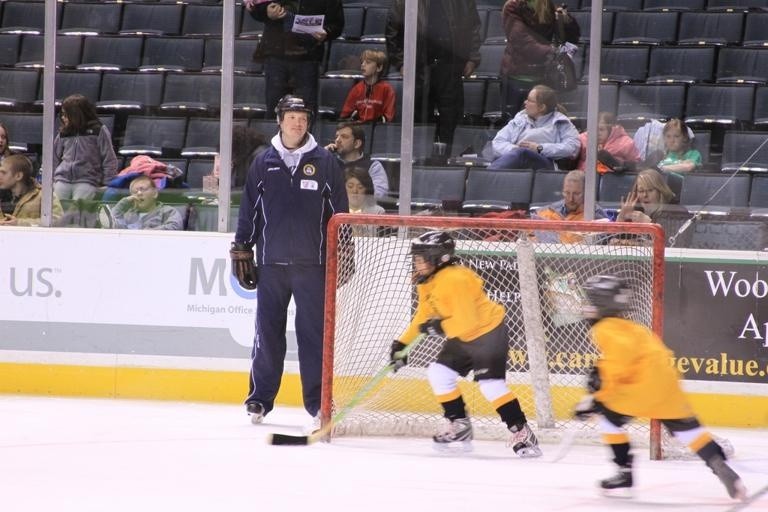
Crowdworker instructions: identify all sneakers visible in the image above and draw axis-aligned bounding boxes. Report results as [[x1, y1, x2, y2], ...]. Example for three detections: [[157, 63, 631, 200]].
[[98, 204, 117, 228], [643, 150, 663, 170], [601, 474, 632, 489], [248, 403, 267, 417], [509, 426, 537, 452], [434, 427, 473, 443], [598, 149, 623, 173]]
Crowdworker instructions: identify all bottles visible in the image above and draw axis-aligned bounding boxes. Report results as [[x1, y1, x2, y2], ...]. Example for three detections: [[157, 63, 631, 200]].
[[37, 164, 43, 186]]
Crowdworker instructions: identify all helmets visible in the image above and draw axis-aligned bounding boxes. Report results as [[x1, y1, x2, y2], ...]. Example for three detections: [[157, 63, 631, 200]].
[[274, 94, 313, 112], [411, 230, 455, 269], [584, 274, 629, 316]]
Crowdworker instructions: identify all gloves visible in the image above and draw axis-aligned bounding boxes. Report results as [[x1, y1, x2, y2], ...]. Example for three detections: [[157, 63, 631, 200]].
[[390, 340, 406, 371], [419, 319, 444, 336], [230, 249, 257, 289]]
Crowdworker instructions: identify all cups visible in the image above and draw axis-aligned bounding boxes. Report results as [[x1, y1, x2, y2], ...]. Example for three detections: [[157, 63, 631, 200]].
[[433, 142, 447, 156]]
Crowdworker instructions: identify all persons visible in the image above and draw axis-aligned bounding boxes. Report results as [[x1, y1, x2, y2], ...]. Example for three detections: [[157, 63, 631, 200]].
[[1, 95, 185, 230], [391, 231, 541, 456], [246, 1, 396, 238], [230, 94, 354, 424], [574, 276, 768, 507], [383, 2, 577, 157], [487, 85, 700, 246]]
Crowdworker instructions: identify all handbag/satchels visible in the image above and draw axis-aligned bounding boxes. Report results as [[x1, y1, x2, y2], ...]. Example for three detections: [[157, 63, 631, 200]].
[[545, 11, 577, 91]]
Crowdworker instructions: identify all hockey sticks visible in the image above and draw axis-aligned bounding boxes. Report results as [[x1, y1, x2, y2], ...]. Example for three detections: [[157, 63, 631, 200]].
[[269, 333, 428, 444]]
